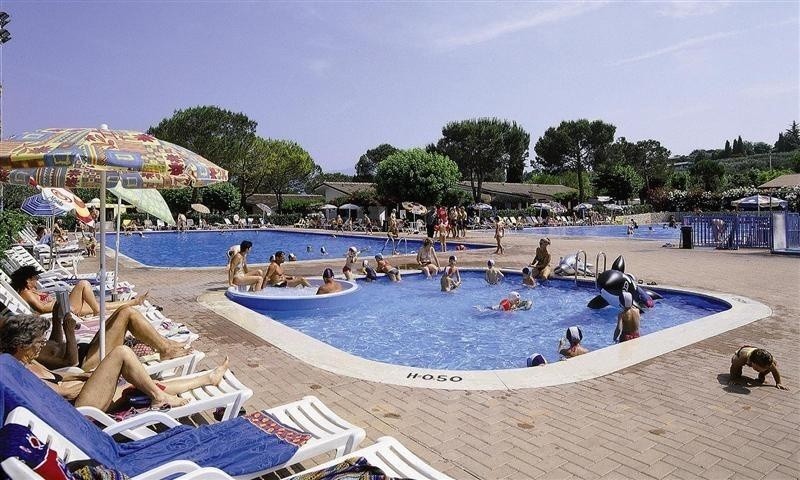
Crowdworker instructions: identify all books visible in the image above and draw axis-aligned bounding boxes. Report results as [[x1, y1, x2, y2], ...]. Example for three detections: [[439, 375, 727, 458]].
[[53, 290, 74, 318]]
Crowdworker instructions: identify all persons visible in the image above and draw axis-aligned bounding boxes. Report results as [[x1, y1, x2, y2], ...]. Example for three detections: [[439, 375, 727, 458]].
[[729, 341, 792, 391], [28, 299, 197, 371], [121, 189, 716, 376], [1, 312, 229, 417]]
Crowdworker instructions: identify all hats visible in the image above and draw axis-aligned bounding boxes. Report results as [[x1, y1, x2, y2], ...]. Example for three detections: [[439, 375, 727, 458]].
[[349, 247, 357, 253], [526, 353, 546, 367], [566, 326, 583, 343], [618, 291, 633, 307], [375, 253, 383, 259], [443, 266, 452, 276], [487, 260, 495, 266], [11, 266, 44, 281], [323, 268, 333, 278]]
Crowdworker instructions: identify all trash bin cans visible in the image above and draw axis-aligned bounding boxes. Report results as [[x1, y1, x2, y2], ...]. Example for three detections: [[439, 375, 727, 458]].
[[383, 220, 388, 231], [680, 227, 692, 249]]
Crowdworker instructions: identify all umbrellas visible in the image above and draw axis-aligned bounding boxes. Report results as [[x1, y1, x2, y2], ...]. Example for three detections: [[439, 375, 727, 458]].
[[731, 194, 789, 216]]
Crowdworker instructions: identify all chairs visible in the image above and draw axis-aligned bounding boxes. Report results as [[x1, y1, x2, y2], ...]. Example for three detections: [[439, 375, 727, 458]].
[[0, 241, 254, 441], [15, 216, 87, 275], [0, 350, 453, 479], [467, 212, 608, 230]]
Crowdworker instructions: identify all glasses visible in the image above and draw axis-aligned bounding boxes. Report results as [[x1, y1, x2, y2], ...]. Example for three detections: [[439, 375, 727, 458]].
[[36, 337, 47, 348]]
